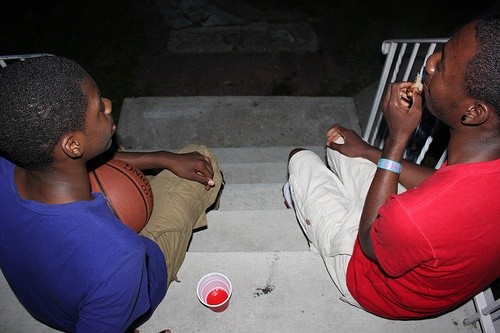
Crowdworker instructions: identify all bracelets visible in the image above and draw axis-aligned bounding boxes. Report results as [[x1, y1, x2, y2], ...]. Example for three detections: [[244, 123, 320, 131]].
[[377, 158, 403, 175]]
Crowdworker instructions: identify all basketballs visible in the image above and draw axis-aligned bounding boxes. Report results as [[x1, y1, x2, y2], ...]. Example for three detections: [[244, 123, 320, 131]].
[[87, 158, 154, 234]]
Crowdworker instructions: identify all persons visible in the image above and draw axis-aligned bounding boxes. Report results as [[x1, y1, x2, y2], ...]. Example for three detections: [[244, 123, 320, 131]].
[[0, 56, 223, 333], [283, 16, 500, 321]]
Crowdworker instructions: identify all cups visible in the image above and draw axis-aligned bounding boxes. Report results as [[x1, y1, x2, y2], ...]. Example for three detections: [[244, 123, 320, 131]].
[[196, 273, 233, 312]]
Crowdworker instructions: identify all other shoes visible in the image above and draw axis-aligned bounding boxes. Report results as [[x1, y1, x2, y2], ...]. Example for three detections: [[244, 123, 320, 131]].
[[283, 181, 293, 210]]
